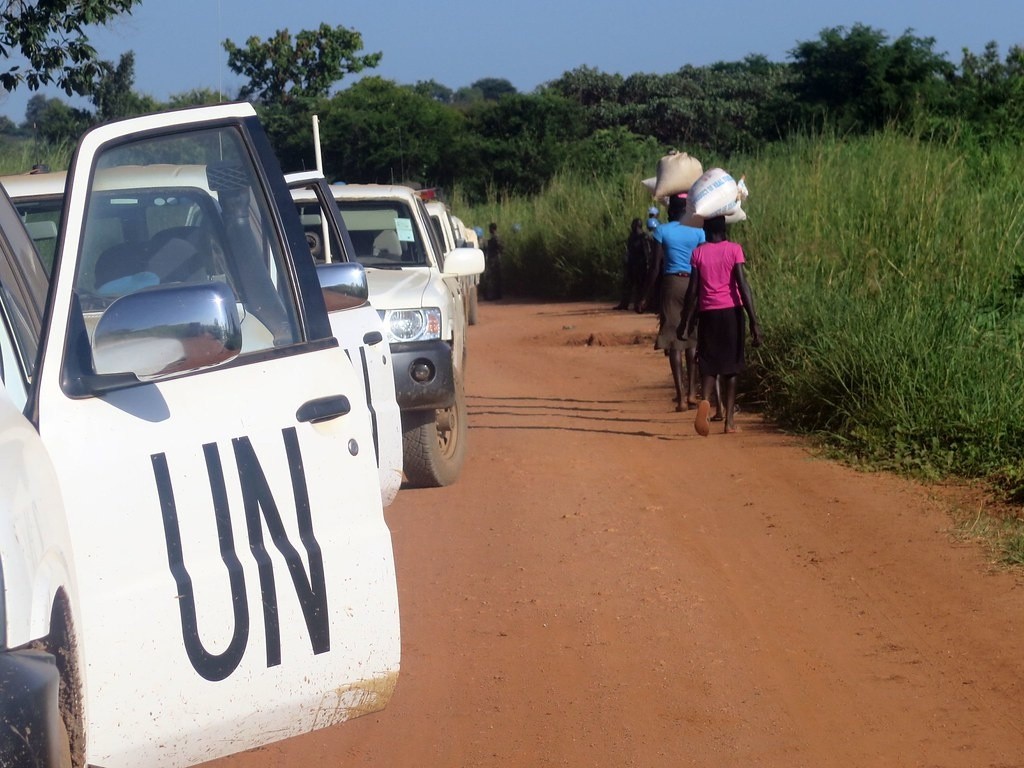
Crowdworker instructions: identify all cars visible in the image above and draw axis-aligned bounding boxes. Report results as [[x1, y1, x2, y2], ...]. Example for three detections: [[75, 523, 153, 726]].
[[0, 163, 404, 509], [0, 101, 401, 768], [185, 184, 486, 487], [424, 201, 480, 326]]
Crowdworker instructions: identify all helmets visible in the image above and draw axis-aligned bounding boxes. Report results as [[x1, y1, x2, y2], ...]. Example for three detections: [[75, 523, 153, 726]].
[[473, 227, 483, 236], [649, 207, 659, 214], [647, 218, 659, 227]]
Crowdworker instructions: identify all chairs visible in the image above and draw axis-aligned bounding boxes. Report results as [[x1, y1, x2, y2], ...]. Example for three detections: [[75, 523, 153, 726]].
[[370, 230, 403, 258], [95, 225, 220, 290]]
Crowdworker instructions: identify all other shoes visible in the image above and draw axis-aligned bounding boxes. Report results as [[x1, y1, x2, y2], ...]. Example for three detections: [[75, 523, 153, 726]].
[[676, 407, 688, 412], [710, 415, 723, 421], [688, 405, 697, 409], [694, 400, 710, 436], [725, 426, 742, 432]]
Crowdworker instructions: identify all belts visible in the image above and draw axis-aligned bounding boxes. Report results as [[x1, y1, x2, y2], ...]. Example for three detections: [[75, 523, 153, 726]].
[[667, 273, 691, 277]]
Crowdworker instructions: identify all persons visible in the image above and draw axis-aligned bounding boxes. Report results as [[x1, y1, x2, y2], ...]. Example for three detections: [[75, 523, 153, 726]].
[[474, 222, 504, 300], [613, 191, 762, 437]]
